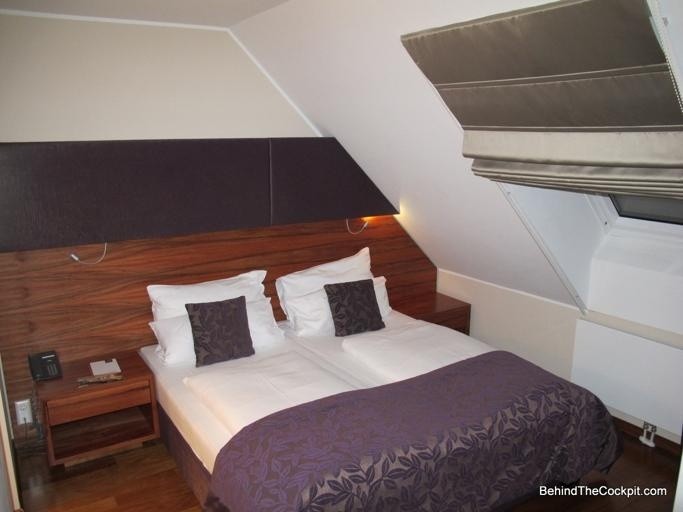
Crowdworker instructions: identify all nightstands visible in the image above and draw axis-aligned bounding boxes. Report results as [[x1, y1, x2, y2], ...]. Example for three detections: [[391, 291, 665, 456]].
[[33, 346, 162, 475], [390, 292, 474, 336]]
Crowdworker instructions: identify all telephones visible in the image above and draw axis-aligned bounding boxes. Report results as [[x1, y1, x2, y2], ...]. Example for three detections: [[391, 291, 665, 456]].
[[28, 350, 62, 382]]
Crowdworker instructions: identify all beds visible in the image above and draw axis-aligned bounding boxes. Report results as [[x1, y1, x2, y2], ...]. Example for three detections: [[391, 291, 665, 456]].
[[135, 300, 630, 511]]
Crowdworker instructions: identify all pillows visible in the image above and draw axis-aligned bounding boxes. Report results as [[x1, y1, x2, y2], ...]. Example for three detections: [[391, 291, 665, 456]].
[[146, 293, 288, 365], [273, 245, 376, 323], [141, 262, 267, 355], [177, 293, 257, 367], [280, 275, 391, 339], [321, 277, 388, 338]]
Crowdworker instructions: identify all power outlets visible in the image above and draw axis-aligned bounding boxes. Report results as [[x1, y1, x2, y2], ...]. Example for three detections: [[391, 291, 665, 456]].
[[13, 398, 33, 428]]
